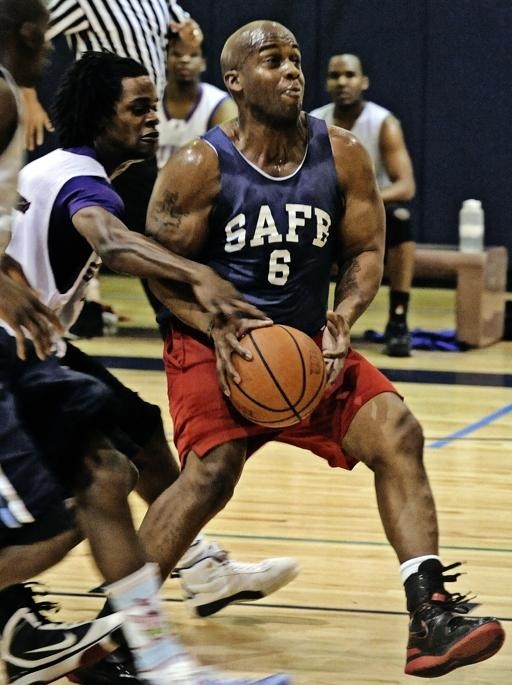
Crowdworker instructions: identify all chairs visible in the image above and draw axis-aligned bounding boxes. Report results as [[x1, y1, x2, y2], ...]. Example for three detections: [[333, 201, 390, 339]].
[[171, 547, 299, 615], [0, 582, 288, 684], [383, 322, 412, 357], [68, 302, 103, 337]]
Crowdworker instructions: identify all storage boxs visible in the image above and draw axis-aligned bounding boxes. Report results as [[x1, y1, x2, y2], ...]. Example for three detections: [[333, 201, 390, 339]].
[[325, 240, 510, 350]]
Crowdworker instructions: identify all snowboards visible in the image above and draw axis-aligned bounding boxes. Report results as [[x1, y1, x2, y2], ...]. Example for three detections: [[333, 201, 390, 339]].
[[224, 323, 325, 427]]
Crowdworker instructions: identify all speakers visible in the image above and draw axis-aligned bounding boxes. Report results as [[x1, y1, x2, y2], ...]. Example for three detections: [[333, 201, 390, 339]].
[[403, 559, 505, 679]]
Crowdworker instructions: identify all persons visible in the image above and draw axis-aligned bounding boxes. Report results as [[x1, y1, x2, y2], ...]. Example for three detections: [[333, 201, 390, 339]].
[[308, 52, 419, 357], [151, 21, 239, 170], [1, 1, 299, 685], [67, 21, 506, 682]]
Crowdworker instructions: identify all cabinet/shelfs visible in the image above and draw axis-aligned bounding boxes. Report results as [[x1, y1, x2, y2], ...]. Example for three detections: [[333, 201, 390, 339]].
[[457, 199, 485, 254]]
